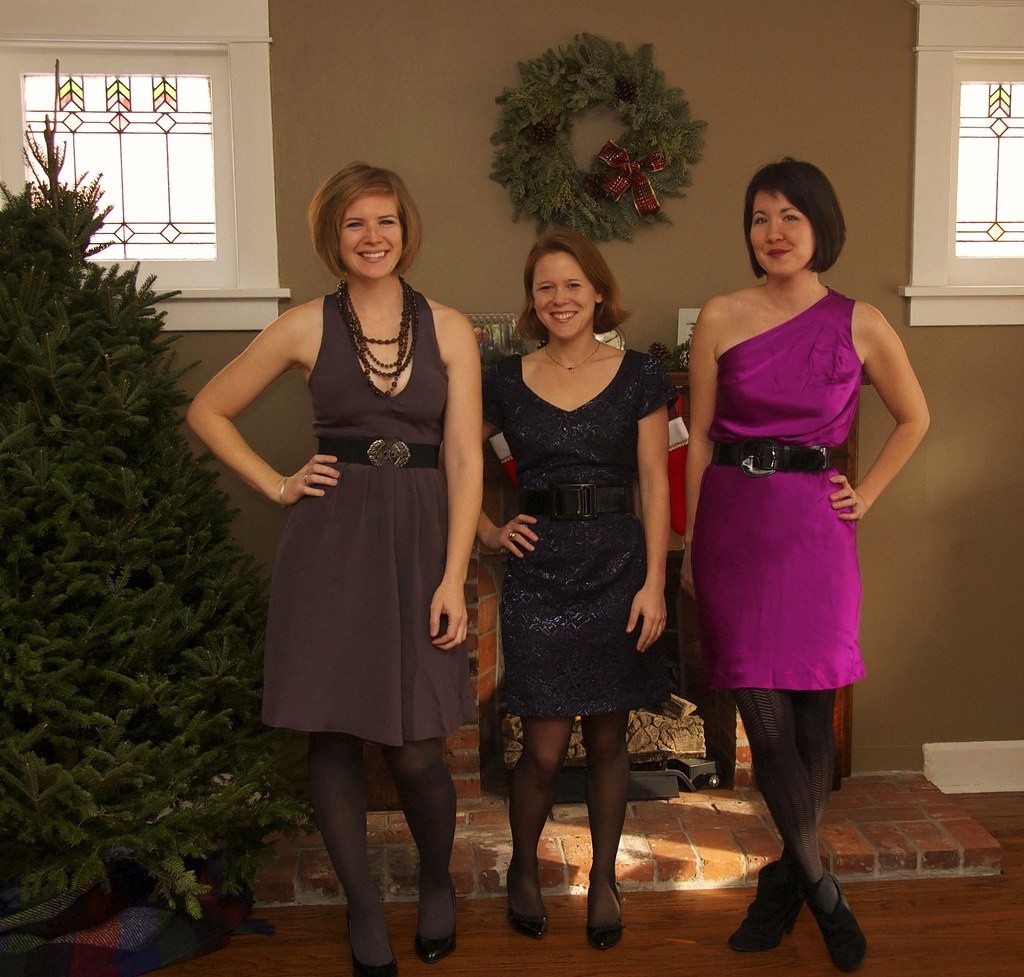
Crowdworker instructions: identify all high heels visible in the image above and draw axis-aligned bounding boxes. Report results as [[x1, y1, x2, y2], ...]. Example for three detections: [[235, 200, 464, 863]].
[[729, 861, 867, 972]]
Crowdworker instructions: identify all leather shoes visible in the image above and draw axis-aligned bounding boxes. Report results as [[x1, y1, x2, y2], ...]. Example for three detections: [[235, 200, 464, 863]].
[[587, 881, 622, 948], [416, 875, 457, 963], [508, 870, 547, 938], [347, 908, 397, 977]]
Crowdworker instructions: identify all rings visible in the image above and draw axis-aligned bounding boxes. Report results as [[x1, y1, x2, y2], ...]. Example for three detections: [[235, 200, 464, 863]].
[[304, 475, 311, 486], [509, 532, 516, 541]]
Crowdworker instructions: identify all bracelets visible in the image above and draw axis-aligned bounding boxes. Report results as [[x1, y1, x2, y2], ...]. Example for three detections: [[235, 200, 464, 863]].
[[280, 476, 288, 510]]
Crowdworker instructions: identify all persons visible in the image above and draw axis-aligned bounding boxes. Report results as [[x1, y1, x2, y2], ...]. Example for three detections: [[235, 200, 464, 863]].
[[681, 158, 930, 970], [187, 162, 484, 977], [477, 229, 672, 952]]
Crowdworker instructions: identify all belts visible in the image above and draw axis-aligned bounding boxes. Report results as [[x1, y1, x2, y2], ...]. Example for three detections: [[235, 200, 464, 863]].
[[711, 439, 830, 477], [519, 485, 634, 516], [318, 435, 440, 471]]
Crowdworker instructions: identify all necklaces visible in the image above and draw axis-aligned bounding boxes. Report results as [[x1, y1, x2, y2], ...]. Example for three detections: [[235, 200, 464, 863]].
[[545, 339, 600, 372], [338, 276, 421, 397]]
